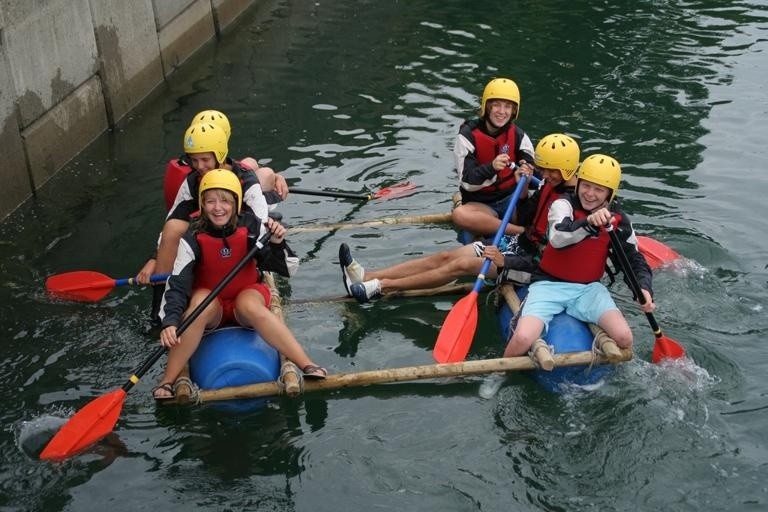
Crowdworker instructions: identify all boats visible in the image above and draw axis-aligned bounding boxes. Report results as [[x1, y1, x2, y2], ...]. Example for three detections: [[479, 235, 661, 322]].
[[157, 190, 632, 414]]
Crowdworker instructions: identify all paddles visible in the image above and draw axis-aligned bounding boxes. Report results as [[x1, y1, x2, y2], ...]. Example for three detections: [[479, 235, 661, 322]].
[[603, 220, 685, 361], [48, 272, 176, 300], [498, 146, 680, 270], [40, 221, 276, 458], [433, 163, 535, 362]]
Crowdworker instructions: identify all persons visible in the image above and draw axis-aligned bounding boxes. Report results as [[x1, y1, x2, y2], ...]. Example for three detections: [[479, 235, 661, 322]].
[[338, 131, 583, 308], [475, 152, 657, 399], [449, 77, 544, 238], [135, 110, 327, 401]]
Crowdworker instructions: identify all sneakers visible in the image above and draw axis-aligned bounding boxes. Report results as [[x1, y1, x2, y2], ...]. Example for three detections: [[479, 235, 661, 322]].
[[479, 375, 508, 398], [339, 243, 364, 296], [350, 279, 381, 302]]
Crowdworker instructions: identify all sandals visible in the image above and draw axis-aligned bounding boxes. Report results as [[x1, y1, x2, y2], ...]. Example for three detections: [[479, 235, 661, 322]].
[[302, 365, 328, 378], [151, 383, 174, 399]]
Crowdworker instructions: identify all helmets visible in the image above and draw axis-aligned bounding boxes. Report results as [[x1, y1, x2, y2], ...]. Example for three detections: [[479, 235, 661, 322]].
[[574, 155, 622, 204], [534, 134, 580, 180], [479, 79, 521, 120], [184, 110, 231, 164], [199, 169, 242, 214]]
